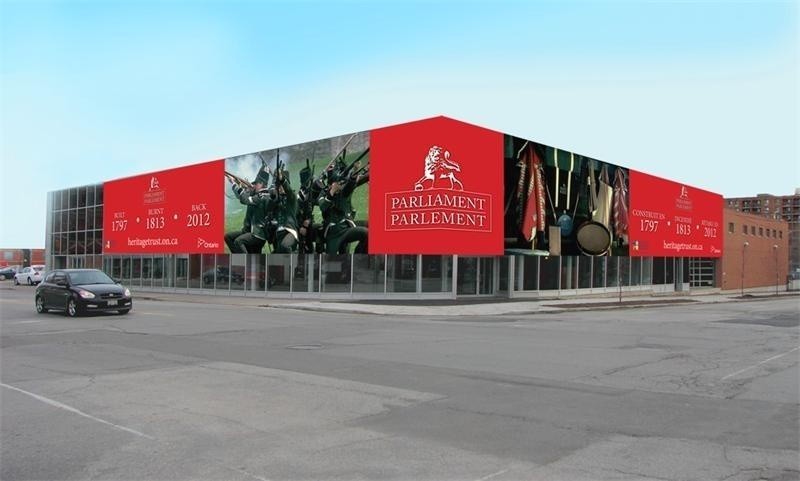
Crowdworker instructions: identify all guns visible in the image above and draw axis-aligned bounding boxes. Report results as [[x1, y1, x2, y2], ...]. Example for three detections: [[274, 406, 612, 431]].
[[299, 165, 315, 256], [319, 132, 369, 200], [224, 170, 255, 194], [256, 148, 284, 203]]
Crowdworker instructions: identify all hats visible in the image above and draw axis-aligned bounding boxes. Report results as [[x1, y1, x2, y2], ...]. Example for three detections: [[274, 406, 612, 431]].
[[327, 166, 345, 185], [298, 167, 314, 186], [281, 170, 291, 184], [249, 165, 271, 187]]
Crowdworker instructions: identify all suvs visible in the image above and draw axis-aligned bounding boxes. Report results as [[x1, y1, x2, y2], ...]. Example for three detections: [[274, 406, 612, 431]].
[[324, 258, 351, 284], [790, 268, 800, 280]]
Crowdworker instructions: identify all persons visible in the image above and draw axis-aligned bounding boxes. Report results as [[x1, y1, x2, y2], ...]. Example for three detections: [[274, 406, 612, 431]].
[[223, 155, 369, 253]]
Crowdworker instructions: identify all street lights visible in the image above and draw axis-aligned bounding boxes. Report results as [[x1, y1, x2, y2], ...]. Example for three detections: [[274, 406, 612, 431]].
[[773, 243, 779, 295], [741, 242, 749, 295]]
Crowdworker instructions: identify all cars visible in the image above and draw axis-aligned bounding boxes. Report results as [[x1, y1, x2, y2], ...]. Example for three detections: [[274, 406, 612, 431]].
[[1, 265, 133, 317], [234, 264, 274, 290], [204, 266, 244, 286]]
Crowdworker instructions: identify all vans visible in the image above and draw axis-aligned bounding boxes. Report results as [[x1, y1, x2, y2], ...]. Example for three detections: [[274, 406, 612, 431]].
[[294, 254, 329, 281]]
